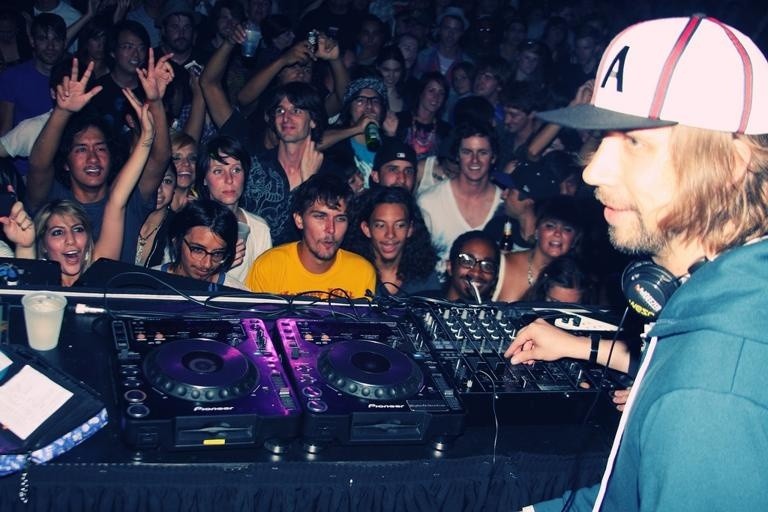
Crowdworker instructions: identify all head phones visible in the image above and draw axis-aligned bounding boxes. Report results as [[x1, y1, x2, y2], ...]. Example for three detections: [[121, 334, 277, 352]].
[[620, 225, 710, 319]]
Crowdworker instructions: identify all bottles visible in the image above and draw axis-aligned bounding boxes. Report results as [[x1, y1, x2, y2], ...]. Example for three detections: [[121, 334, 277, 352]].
[[364, 123, 379, 150], [500, 216, 514, 251]]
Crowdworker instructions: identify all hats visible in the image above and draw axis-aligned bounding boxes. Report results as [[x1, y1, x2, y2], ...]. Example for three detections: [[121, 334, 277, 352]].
[[492, 163, 560, 203], [435, 7, 471, 33], [154, 1, 202, 30], [343, 78, 387, 101], [532, 12, 768, 137], [369, 141, 417, 189]]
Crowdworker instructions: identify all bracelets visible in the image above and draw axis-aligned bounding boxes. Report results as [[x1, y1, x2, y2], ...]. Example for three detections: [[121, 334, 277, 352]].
[[586, 330, 603, 365]]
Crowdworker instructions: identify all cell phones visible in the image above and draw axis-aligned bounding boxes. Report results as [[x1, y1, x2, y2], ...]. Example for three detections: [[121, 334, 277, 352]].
[[306, 32, 318, 54]]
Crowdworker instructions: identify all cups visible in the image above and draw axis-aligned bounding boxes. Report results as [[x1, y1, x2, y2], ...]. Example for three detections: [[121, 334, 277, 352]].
[[21, 289, 68, 350], [236, 221, 250, 242], [242, 30, 261, 58]]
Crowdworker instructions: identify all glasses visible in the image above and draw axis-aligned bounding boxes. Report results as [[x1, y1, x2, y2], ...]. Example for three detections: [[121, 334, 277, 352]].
[[455, 252, 497, 275], [352, 95, 382, 109], [182, 237, 230, 265]]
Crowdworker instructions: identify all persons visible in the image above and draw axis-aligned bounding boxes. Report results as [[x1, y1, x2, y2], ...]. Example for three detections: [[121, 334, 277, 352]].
[[502, 317, 642, 413], [2, 1, 615, 305], [500, 14, 767, 510]]
